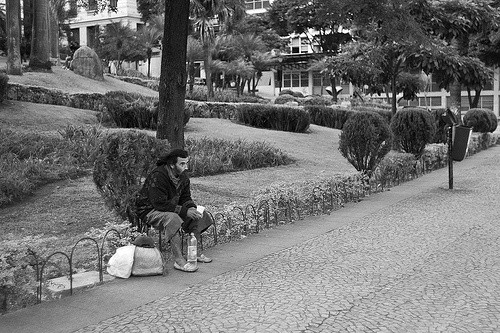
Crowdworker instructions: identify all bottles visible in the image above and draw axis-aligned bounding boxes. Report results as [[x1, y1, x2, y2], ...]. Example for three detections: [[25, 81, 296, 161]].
[[187, 232, 198, 266]]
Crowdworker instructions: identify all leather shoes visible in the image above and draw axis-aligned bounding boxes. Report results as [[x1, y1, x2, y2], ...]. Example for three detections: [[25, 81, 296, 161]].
[[174, 262, 199, 272], [197, 254, 211, 263]]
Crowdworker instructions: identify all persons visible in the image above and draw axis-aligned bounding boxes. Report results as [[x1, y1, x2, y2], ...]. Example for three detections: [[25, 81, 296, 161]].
[[136, 149, 212, 272], [63, 41, 79, 70]]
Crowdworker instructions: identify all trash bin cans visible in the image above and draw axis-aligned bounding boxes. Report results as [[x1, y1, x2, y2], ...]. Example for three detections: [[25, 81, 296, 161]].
[[447, 125, 472, 190]]
